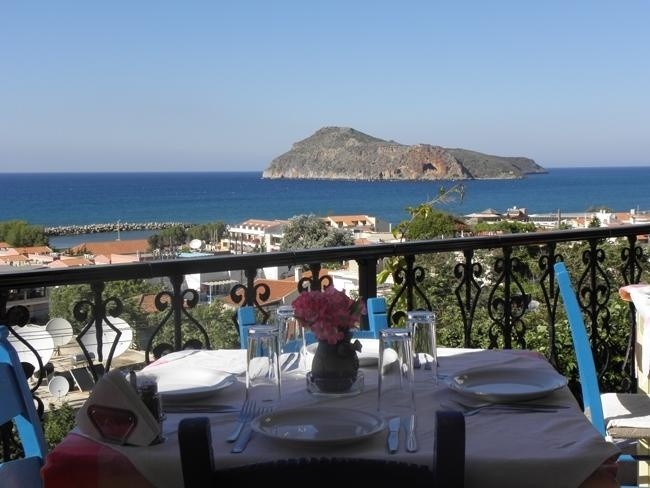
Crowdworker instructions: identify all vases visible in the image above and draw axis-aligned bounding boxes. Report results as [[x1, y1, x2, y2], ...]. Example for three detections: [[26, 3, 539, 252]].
[[312, 333, 359, 390]]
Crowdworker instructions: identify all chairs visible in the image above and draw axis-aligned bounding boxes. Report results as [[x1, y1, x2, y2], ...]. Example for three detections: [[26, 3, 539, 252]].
[[0, 319, 47, 475], [0, 365, 42, 487], [238, 298, 387, 355], [545, 249, 650, 486]]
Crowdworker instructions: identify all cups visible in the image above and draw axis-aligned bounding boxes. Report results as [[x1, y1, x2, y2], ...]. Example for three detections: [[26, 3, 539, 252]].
[[247, 324, 280, 404], [406, 311, 438, 386], [274, 305, 311, 382], [135, 373, 164, 443], [379, 329, 416, 412]]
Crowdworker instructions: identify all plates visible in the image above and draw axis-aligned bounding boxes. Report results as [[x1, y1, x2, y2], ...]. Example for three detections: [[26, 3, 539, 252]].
[[148, 368, 235, 402], [249, 405, 387, 448], [299, 338, 381, 368], [448, 369, 568, 403]]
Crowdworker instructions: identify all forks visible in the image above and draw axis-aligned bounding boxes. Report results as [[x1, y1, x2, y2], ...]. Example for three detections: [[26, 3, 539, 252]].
[[439, 399, 571, 416], [225, 397, 276, 455]]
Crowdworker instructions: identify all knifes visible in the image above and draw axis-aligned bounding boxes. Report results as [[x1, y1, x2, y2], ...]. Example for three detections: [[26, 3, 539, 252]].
[[387, 414, 401, 453], [402, 413, 418, 453], [163, 403, 240, 414]]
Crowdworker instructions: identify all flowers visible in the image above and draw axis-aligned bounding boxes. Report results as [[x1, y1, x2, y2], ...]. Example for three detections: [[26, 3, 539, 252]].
[[290, 287, 367, 340]]
